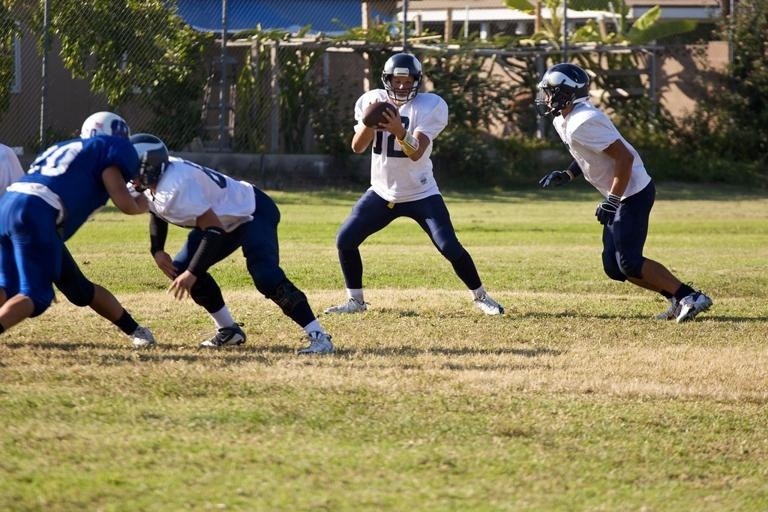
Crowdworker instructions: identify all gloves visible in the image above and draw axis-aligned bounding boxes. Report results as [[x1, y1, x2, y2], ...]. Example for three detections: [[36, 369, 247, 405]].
[[539, 171, 570, 190], [595, 193, 621, 227]]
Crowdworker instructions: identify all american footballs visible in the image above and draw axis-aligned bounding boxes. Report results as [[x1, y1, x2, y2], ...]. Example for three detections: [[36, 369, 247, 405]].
[[361, 102, 396, 131]]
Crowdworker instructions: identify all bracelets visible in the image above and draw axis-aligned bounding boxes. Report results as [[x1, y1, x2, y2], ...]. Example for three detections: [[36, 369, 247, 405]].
[[395, 132, 419, 157]]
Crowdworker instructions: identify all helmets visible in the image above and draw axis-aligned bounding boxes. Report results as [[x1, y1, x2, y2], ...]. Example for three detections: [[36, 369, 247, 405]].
[[129, 133, 170, 196], [381, 53, 423, 107], [534, 63, 590, 118], [80, 111, 129, 137]]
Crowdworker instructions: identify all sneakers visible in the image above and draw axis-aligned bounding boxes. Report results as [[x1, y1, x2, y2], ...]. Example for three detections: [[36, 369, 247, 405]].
[[655, 281, 713, 324], [129, 296, 368, 354], [473, 289, 505, 315]]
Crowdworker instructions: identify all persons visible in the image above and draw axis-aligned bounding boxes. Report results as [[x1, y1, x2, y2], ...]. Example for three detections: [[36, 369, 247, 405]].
[[324, 52, 504, 317], [534, 63, 713, 324], [129, 133, 334, 355], [0, 111, 150, 335], [0, 145, 155, 350]]
